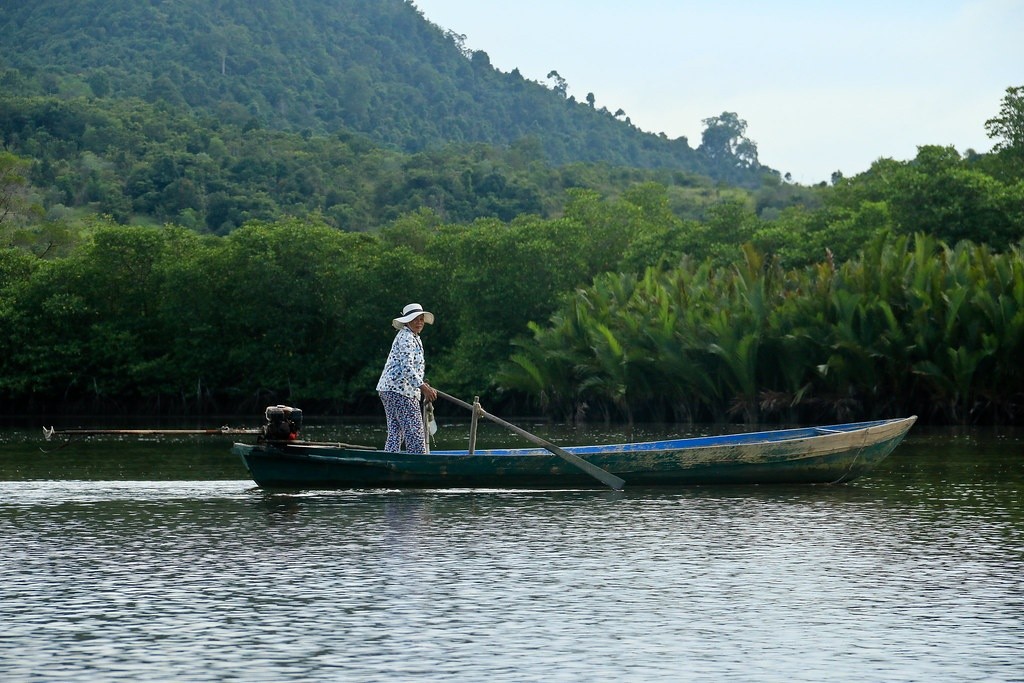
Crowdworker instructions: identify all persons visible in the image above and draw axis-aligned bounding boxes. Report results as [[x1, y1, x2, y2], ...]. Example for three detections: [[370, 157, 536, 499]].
[[376, 304, 438, 454]]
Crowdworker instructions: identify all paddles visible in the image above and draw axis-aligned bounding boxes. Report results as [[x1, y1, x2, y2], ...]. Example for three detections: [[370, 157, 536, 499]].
[[426, 387, 627, 490]]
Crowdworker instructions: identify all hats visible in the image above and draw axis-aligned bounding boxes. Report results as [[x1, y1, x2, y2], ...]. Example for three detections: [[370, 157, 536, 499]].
[[392, 303, 434, 330]]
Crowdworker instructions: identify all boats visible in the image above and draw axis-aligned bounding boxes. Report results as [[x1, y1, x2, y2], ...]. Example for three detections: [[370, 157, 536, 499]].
[[233, 404, 919, 490]]
